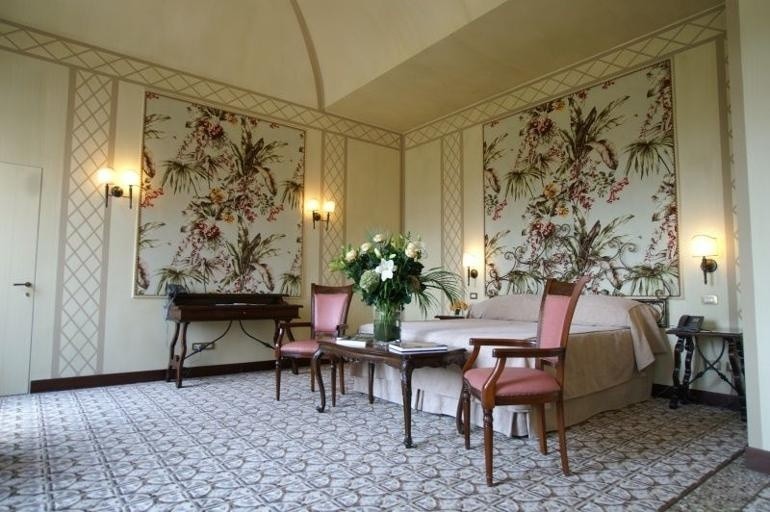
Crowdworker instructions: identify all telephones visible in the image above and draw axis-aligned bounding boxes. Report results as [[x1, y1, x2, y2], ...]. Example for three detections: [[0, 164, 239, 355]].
[[677, 315, 704, 330]]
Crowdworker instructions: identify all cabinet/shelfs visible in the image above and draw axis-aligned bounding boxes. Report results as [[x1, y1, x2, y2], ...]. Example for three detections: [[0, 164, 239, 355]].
[[164, 292, 303, 390]]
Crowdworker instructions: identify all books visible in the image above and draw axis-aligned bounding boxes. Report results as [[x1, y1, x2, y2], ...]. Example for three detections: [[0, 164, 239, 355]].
[[336, 331, 377, 349], [389, 340, 450, 352]]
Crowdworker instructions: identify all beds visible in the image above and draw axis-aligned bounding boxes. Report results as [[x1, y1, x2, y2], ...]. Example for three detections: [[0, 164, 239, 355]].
[[353, 290, 662, 436]]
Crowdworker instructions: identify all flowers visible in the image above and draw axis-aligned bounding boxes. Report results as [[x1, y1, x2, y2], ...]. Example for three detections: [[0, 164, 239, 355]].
[[327, 230, 466, 318]]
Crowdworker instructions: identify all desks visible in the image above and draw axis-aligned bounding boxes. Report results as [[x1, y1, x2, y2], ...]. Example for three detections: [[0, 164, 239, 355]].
[[665, 330, 749, 420], [310, 337, 469, 449]]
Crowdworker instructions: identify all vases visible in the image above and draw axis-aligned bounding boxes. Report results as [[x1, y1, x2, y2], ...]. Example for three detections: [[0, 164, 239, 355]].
[[372, 311, 402, 346]]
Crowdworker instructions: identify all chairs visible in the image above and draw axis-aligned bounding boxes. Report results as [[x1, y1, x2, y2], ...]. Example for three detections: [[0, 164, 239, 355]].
[[273, 281, 354, 406], [458, 276, 590, 486]]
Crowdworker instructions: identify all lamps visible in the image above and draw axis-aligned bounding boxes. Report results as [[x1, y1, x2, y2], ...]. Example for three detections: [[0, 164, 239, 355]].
[[690, 235, 723, 284], [307, 197, 335, 229], [94, 164, 140, 209], [462, 251, 479, 287]]
